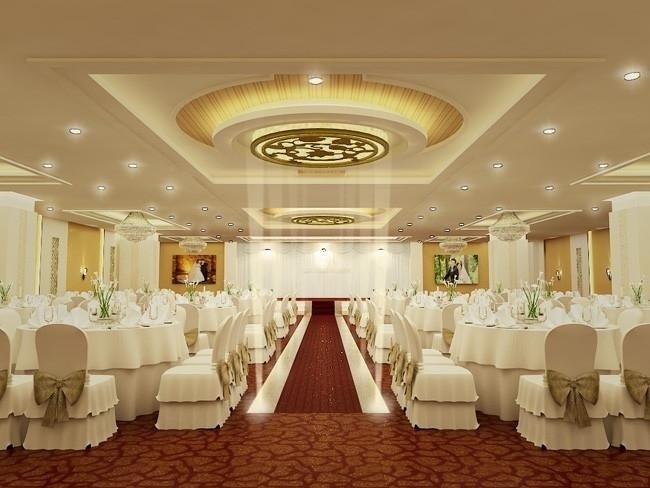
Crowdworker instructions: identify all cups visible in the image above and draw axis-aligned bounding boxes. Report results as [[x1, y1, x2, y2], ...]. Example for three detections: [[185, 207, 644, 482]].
[[415, 294, 422, 303], [27, 295, 33, 304], [476, 301, 609, 325], [37, 300, 160, 323]]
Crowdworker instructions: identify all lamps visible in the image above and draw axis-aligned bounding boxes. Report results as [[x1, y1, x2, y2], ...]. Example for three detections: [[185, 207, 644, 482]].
[[438, 233, 468, 258], [260, 206, 385, 227], [176, 235, 207, 254], [113, 211, 156, 246], [489, 211, 531, 242], [170, 73, 463, 170]]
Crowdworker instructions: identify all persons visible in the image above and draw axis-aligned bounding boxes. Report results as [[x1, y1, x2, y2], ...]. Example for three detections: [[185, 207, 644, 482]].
[[200, 259, 208, 283], [456, 260, 471, 284], [442, 258, 459, 282], [185, 258, 201, 286]]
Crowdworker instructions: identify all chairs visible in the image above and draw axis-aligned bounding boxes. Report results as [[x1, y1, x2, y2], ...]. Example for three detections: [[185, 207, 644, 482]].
[[1, 329, 34, 453], [512, 322, 612, 451], [22, 323, 120, 451], [387, 307, 481, 433], [600, 323, 649, 451], [346, 282, 650, 365], [153, 307, 251, 432], [1, 285, 299, 366]]
